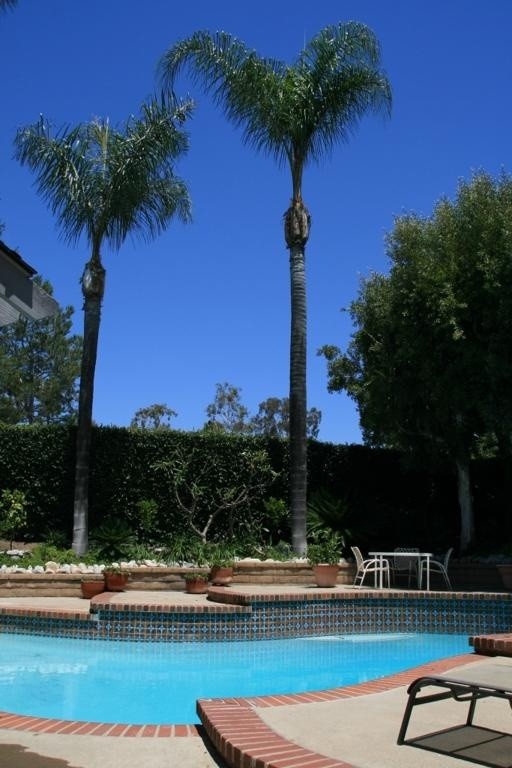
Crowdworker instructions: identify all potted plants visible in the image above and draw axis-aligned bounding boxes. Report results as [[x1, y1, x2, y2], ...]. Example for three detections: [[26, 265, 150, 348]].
[[182, 559, 235, 592], [308, 528, 345, 588], [104, 567, 131, 591]]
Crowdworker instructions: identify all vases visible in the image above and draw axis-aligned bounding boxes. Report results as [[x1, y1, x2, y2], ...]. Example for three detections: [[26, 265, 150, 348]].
[[496, 565, 512, 589], [80, 580, 105, 598]]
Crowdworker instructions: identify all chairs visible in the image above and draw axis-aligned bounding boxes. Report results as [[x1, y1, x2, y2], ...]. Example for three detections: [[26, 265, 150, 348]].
[[397, 655, 512, 745], [349, 546, 455, 591]]
